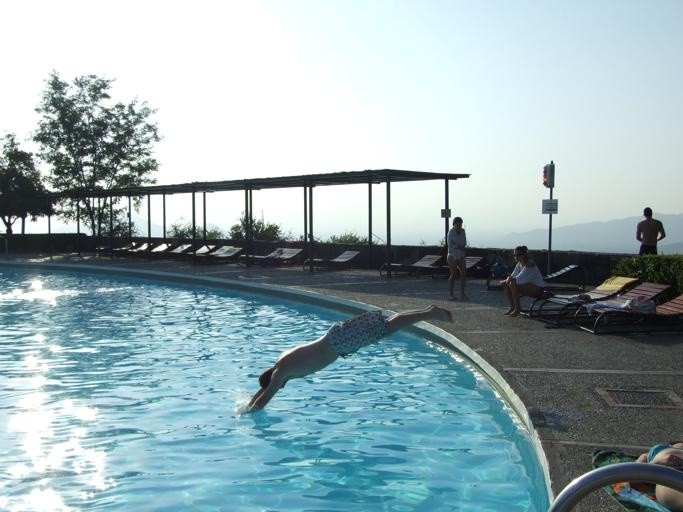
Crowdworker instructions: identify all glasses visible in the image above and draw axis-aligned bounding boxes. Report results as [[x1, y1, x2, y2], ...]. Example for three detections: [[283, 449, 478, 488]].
[[513, 253, 524, 257]]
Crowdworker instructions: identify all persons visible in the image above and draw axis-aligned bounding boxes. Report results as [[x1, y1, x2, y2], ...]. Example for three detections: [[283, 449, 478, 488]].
[[446, 216, 468, 301], [243, 303, 453, 413], [636, 207, 666, 256], [500, 244, 545, 318], [634, 442, 682, 509]]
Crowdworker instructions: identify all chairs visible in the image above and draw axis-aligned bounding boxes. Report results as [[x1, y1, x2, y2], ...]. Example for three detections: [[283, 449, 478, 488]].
[[378, 254, 443, 279], [590, 441, 683, 512], [572, 294, 682, 335], [302, 251, 361, 271], [534, 280, 671, 329], [97, 243, 306, 275], [515, 275, 638, 318], [488, 262, 587, 293], [402, 257, 483, 279]]
